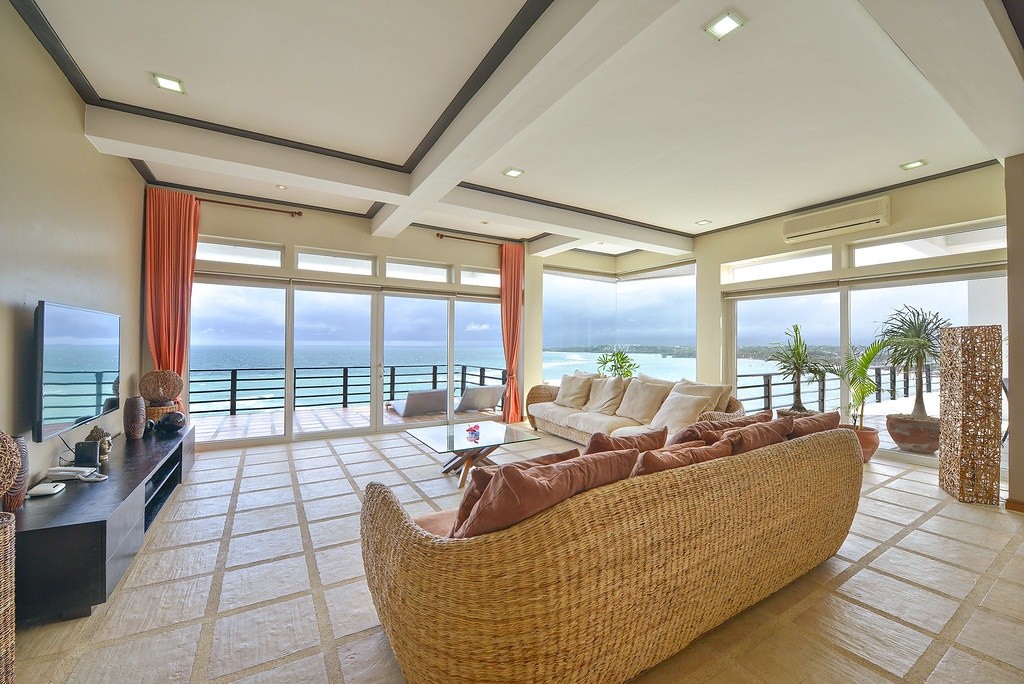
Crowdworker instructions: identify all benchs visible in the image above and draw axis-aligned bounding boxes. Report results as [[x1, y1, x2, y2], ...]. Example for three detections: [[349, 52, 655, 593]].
[[360, 428, 864, 684], [526, 384, 745, 447]]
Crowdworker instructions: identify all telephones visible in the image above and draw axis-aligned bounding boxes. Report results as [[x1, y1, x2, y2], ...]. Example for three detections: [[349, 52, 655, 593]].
[[47, 467, 97, 481]]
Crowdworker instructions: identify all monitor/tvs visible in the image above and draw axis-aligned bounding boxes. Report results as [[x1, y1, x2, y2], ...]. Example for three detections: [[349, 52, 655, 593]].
[[33, 301, 120, 443]]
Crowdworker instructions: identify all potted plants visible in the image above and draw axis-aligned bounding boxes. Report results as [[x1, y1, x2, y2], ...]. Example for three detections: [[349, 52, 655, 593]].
[[873, 304, 952, 455], [808, 335, 898, 464], [764, 325, 826, 420]]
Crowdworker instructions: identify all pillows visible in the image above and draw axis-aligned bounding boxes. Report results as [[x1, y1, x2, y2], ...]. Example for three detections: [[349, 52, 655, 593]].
[[581, 375, 637, 416], [447, 409, 841, 539], [554, 370, 601, 410], [650, 377, 732, 434], [615, 373, 676, 425]]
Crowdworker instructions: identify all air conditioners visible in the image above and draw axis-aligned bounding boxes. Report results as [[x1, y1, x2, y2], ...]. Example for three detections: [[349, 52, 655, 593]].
[[781, 196, 890, 245]]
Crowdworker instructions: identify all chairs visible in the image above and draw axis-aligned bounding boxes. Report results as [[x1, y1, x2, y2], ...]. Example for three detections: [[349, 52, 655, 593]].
[[385, 388, 455, 418], [453, 385, 506, 415]]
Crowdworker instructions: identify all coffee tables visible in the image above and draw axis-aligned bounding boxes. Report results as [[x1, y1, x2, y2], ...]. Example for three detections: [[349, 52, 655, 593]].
[[404, 420, 541, 490]]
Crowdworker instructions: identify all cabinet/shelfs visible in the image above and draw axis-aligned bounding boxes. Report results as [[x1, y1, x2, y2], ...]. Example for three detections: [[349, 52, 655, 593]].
[[12, 425, 194, 622]]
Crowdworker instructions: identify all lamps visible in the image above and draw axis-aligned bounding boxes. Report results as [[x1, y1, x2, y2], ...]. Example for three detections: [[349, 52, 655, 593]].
[[151, 73, 186, 95], [702, 10, 746, 43], [503, 166, 525, 179], [899, 159, 928, 171]]
